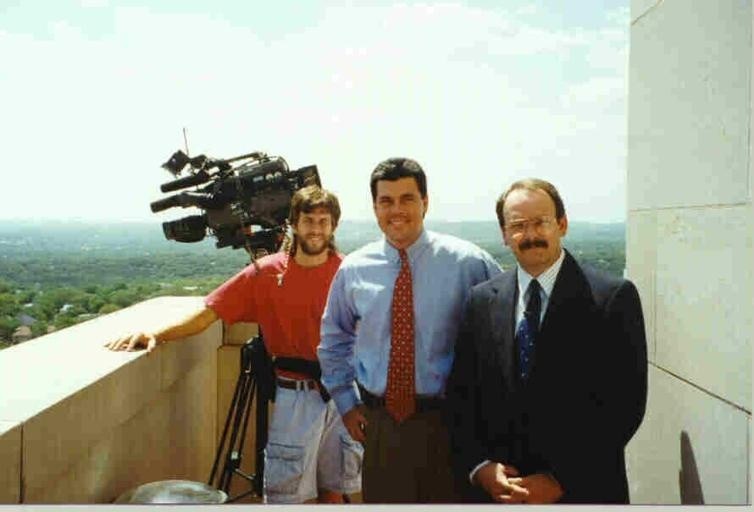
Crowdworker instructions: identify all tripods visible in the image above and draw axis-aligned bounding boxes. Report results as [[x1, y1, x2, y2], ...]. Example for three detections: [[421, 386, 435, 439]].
[[208, 323, 353, 504]]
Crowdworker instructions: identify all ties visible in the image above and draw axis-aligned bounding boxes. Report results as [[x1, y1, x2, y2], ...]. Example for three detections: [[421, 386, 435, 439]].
[[384, 248, 417, 422], [515, 279, 540, 387]]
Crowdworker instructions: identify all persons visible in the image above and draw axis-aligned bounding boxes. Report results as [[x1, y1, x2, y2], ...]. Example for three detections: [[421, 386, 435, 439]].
[[312, 157, 507, 506], [105, 185, 370, 503], [441, 176, 648, 504]]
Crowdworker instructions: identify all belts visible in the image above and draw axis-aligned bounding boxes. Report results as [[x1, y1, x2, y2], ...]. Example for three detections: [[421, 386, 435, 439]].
[[361, 390, 446, 411], [277, 378, 314, 390]]
